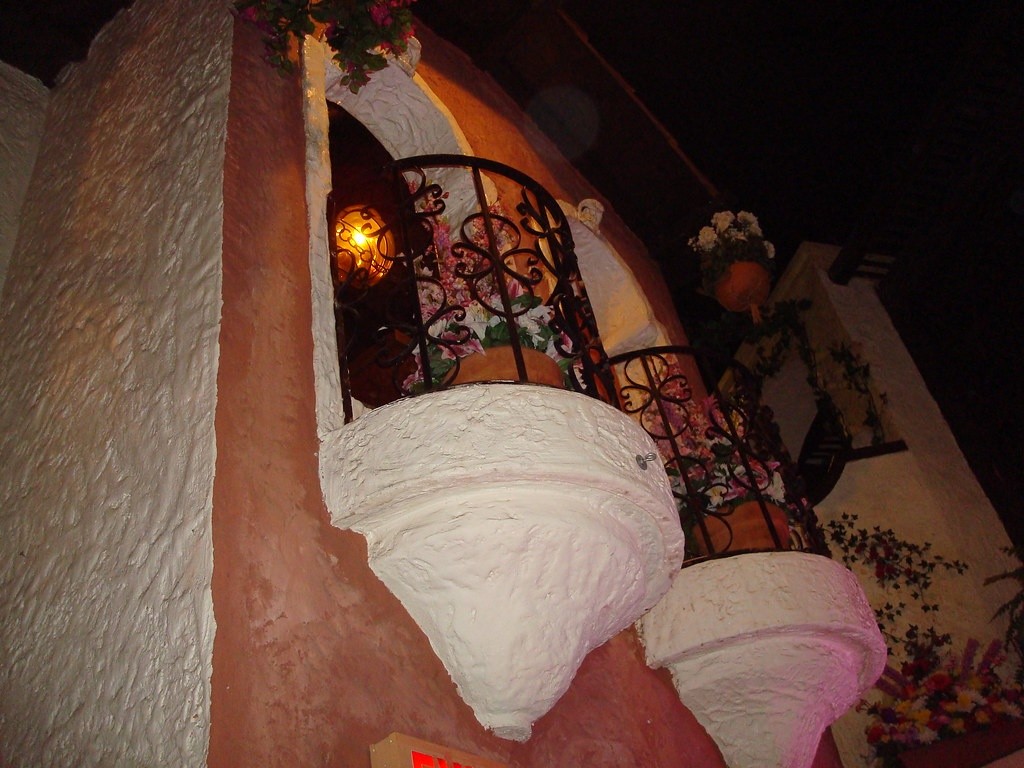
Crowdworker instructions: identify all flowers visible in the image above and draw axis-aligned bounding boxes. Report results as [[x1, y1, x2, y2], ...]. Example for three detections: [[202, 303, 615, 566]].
[[692, 208, 779, 299], [823, 520, 1024, 768], [398, 179, 587, 395], [232, 0, 415, 96], [638, 356, 810, 550]]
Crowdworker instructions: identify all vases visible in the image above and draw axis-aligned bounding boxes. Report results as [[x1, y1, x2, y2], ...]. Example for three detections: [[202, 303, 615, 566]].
[[899, 715, 1024, 768], [693, 502, 789, 559], [442, 346, 564, 388], [715, 261, 772, 325]]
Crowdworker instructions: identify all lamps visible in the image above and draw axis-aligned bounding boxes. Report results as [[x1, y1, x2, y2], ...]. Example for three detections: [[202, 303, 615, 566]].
[[334, 204, 394, 288]]
